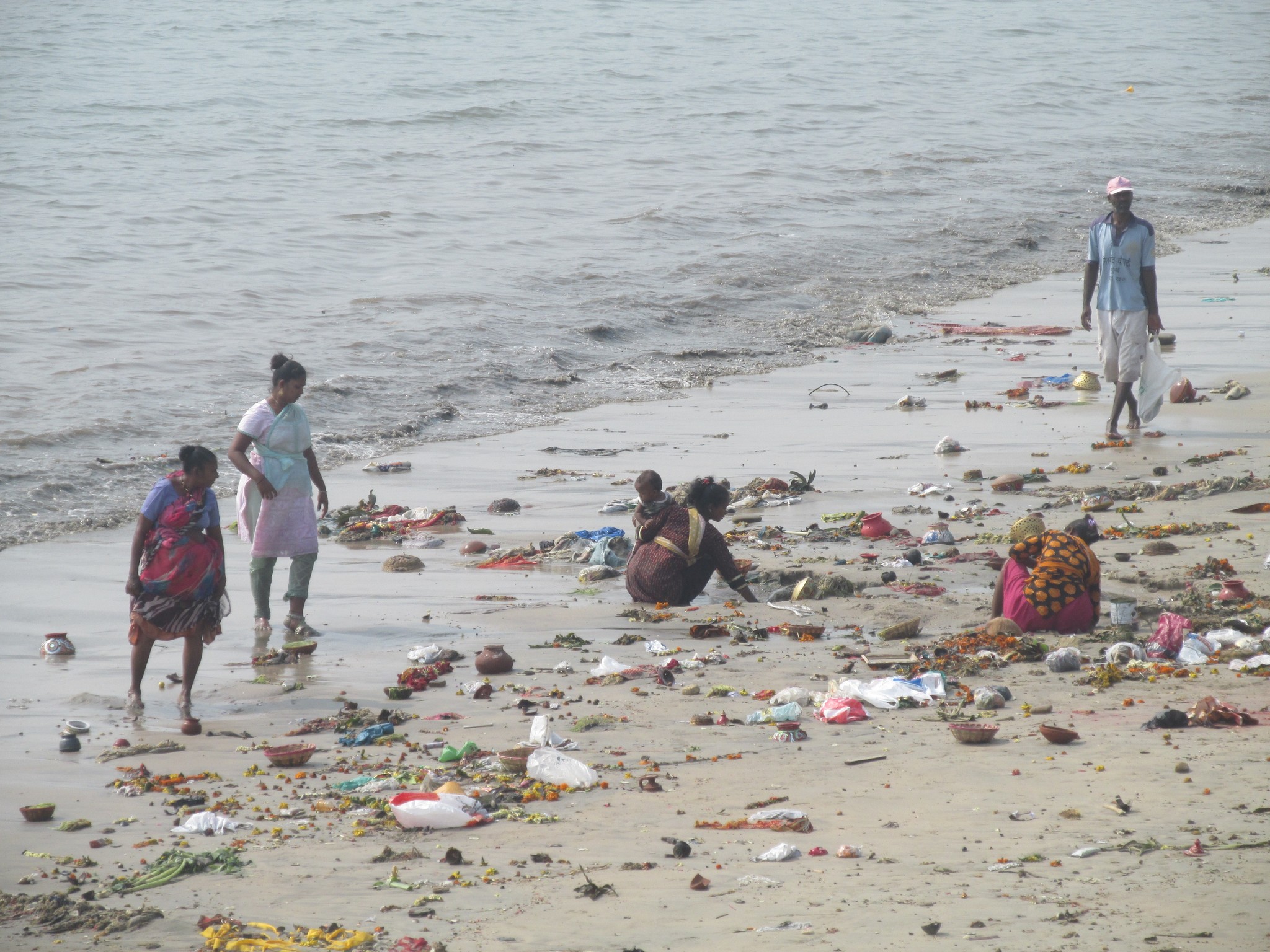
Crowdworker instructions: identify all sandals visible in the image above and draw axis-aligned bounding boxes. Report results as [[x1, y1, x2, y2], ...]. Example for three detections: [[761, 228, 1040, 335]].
[[283, 615, 321, 636]]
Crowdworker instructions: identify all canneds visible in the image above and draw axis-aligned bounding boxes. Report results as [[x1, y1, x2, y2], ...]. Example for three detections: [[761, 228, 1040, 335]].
[[423, 742, 444, 749]]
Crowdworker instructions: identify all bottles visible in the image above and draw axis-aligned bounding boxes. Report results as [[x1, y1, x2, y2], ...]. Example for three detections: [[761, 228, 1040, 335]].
[[1169, 377, 1197, 403], [1082, 486, 1114, 511], [860, 512, 892, 537], [771, 722, 807, 743], [40, 632, 75, 655], [791, 576, 823, 600], [921, 522, 955, 546], [902, 548, 922, 565], [1218, 580, 1250, 600], [474, 644, 513, 674], [180, 717, 201, 736], [716, 710, 730, 725]]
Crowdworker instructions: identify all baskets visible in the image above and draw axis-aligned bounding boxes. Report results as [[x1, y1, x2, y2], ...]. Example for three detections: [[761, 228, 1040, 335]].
[[949, 723, 999, 743], [498, 748, 537, 772], [264, 743, 316, 767]]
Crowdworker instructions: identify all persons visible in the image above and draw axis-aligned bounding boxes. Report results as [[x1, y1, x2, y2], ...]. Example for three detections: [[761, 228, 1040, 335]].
[[228, 354, 328, 636], [126, 445, 231, 705], [991, 513, 1101, 633], [1081, 176, 1165, 440], [635, 469, 679, 528], [625, 476, 760, 606]]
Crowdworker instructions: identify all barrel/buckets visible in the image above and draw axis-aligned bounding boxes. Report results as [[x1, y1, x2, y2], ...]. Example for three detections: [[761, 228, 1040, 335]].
[[1110, 597, 1138, 632]]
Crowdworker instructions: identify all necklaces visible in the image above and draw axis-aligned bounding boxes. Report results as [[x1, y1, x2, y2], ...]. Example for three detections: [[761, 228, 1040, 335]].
[[182, 474, 200, 503]]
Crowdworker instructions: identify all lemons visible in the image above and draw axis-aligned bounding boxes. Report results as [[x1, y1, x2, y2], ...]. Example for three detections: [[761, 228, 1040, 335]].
[[158, 681, 165, 688]]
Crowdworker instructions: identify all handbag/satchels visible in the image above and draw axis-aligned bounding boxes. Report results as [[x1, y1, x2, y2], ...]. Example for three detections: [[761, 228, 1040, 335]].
[[1145, 612, 1192, 659], [527, 748, 600, 790], [390, 792, 489, 829], [1137, 331, 1181, 423]]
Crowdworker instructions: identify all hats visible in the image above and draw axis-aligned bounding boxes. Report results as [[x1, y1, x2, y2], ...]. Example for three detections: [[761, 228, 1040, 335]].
[[1107, 177, 1133, 199]]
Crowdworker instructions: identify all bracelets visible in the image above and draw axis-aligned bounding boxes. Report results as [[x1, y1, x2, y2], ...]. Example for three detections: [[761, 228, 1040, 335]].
[[318, 490, 327, 493], [256, 476, 265, 484], [634, 518, 636, 524]]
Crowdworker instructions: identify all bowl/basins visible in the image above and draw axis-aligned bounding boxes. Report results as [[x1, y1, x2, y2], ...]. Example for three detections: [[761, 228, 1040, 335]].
[[1137, 571, 1147, 577], [1039, 725, 1078, 745], [281, 641, 317, 655], [58, 734, 81, 751], [860, 554, 879, 560], [19, 805, 55, 822], [1115, 553, 1131, 562], [113, 738, 130, 748], [987, 558, 1009, 571], [66, 720, 91, 734]]
